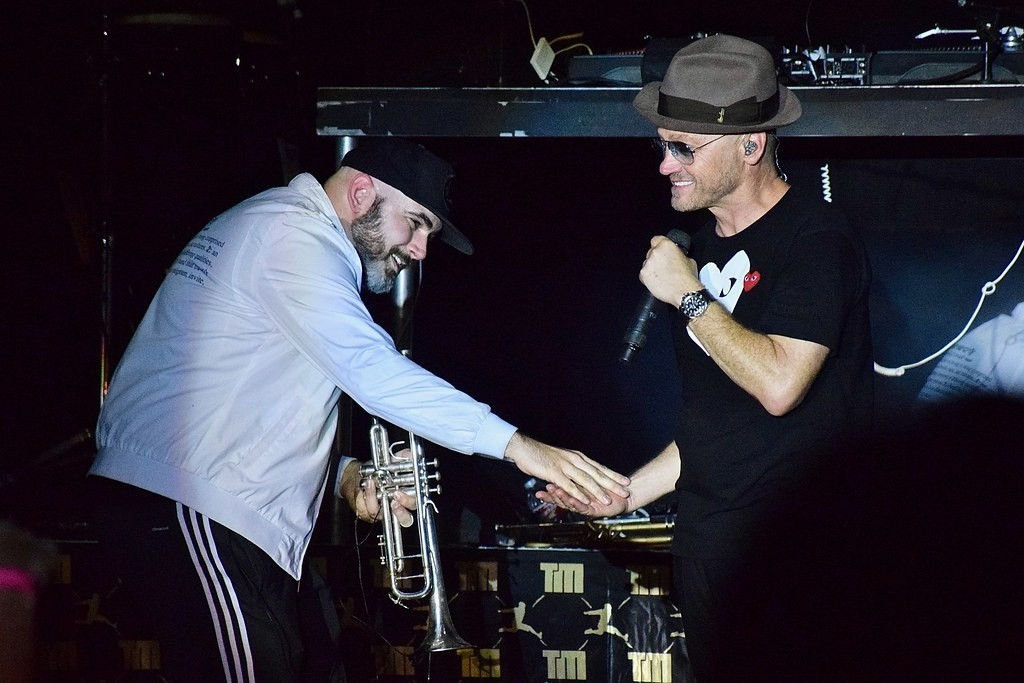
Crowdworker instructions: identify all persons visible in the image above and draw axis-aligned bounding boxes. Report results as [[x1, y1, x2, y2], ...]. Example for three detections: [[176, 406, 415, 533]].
[[82, 135, 632, 683], [535, 33, 891, 683]]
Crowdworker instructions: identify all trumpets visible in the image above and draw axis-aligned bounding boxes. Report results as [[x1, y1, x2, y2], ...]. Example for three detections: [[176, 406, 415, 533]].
[[360, 349, 477, 653]]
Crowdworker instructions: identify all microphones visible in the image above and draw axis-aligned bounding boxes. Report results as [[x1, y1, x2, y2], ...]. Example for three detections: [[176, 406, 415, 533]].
[[616, 227, 694, 366]]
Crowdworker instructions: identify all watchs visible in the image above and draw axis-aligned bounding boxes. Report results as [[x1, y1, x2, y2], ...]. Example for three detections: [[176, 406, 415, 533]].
[[677, 289, 718, 328]]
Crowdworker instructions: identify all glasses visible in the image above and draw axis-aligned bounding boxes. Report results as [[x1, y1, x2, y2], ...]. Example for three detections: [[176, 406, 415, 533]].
[[652, 135, 724, 165]]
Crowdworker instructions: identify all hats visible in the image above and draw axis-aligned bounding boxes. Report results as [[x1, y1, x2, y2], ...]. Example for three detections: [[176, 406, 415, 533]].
[[341, 140, 475, 256], [632, 35, 802, 134]]
